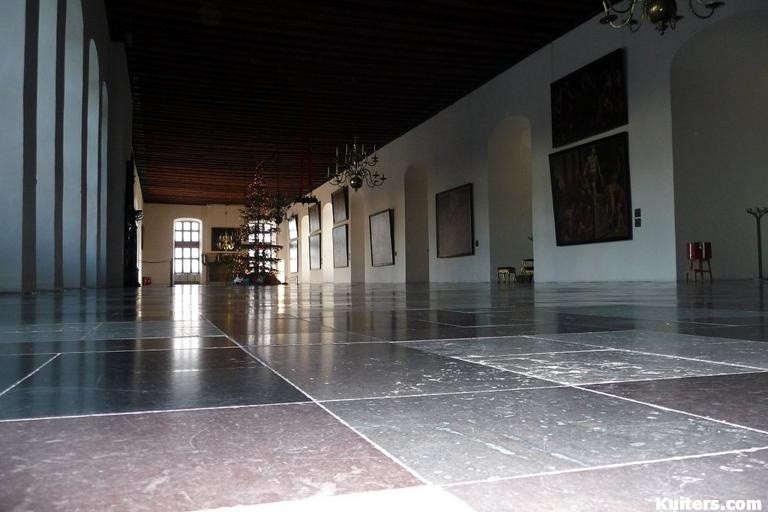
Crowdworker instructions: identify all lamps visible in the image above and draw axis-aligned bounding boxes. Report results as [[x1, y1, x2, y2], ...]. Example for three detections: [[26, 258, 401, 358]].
[[265, 147, 295, 227], [216, 204, 236, 252], [599, 0, 726, 37]]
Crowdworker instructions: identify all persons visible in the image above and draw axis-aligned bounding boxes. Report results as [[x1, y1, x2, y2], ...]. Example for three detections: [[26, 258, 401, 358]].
[[600, 184, 627, 232], [582, 147, 602, 200]]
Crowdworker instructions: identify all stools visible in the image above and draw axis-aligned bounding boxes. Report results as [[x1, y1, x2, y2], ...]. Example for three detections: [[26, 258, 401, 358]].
[[497, 267, 516, 288]]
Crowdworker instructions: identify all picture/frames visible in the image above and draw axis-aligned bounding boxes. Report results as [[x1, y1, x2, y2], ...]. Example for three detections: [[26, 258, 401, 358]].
[[287, 46, 634, 273]]
